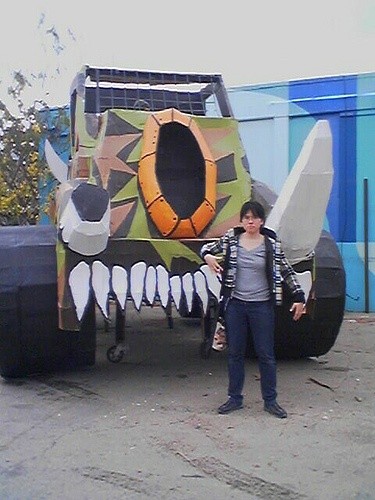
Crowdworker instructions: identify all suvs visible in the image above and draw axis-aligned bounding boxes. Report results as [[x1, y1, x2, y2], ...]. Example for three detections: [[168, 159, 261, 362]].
[[0, 59, 347, 380]]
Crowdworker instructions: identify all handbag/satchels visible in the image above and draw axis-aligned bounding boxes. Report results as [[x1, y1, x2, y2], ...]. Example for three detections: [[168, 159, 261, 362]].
[[210, 312, 228, 352]]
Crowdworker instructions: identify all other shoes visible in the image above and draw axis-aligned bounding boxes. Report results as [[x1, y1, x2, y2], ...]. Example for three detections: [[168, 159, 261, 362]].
[[263, 402, 287, 418], [218, 397, 242, 414]]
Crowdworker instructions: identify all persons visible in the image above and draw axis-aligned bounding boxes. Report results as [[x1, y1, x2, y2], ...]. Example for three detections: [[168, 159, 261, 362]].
[[197, 201, 311, 419]]
